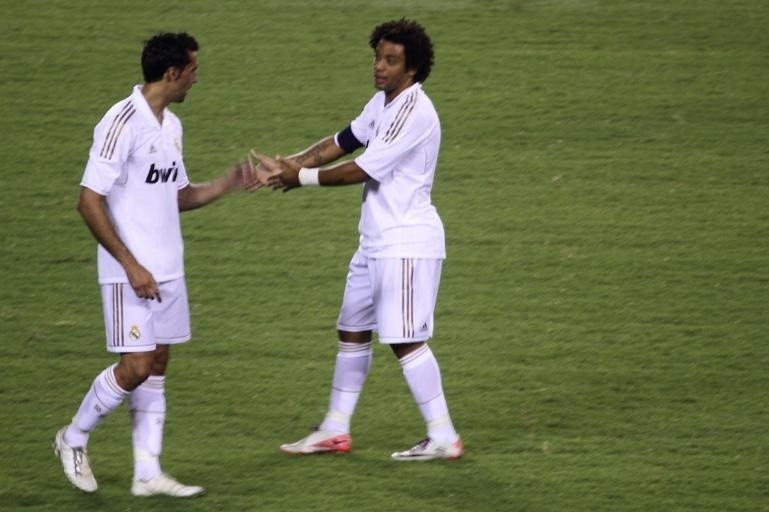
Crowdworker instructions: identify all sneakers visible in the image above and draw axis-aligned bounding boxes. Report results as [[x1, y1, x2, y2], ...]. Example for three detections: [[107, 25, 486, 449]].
[[131, 472, 207, 498], [390, 435, 464, 462], [51, 424, 98, 493], [279, 431, 352, 456]]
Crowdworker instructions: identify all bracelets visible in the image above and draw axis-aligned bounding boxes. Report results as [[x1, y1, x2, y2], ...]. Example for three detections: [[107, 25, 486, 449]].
[[296, 165, 323, 189]]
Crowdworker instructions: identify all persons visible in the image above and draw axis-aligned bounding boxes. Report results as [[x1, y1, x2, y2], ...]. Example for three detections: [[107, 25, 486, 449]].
[[249, 18, 465, 464], [51, 30, 257, 498]]
